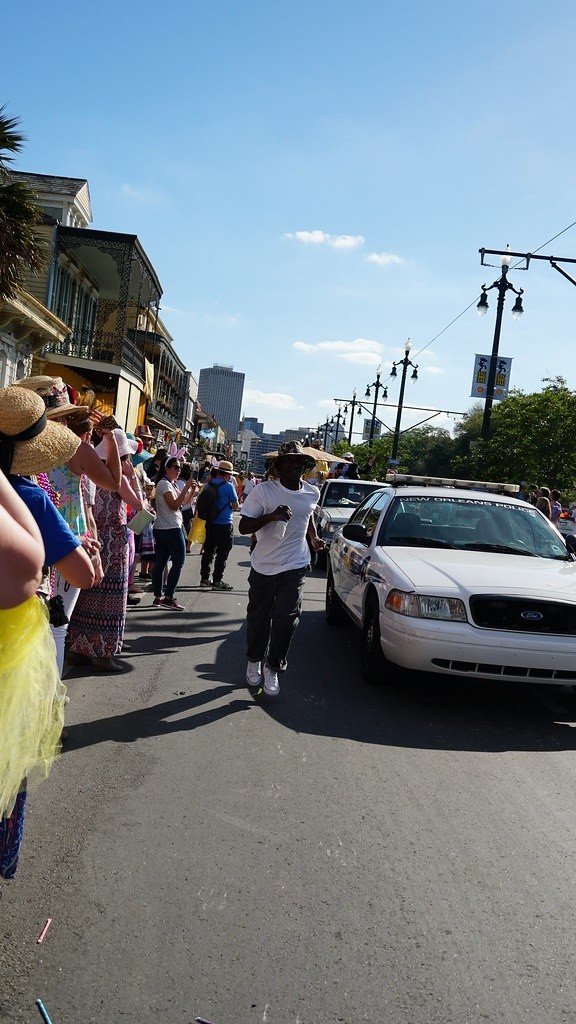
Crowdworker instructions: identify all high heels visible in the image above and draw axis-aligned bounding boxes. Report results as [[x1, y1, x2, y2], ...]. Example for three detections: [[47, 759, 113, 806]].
[[91, 657, 124, 672]]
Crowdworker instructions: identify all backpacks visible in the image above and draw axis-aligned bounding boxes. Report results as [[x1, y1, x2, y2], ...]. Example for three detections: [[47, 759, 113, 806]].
[[196, 480, 230, 520]]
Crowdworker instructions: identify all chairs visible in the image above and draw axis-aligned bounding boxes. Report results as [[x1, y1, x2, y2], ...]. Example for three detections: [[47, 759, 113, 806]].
[[388, 513, 423, 539], [469, 519, 507, 542]]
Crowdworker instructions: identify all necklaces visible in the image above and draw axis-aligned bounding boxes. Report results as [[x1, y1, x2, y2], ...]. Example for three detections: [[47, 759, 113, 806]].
[[38, 472, 59, 597]]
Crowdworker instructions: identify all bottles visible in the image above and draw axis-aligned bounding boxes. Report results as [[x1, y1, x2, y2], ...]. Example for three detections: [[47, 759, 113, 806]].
[[273, 509, 290, 540]]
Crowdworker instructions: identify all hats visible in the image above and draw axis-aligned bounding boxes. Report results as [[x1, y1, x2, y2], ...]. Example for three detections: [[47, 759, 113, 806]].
[[342, 452, 354, 458], [0, 374, 158, 476], [265, 440, 316, 477], [214, 460, 239, 474]]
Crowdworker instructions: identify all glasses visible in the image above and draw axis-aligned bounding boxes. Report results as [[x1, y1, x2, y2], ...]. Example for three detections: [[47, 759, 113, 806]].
[[172, 465, 183, 471]]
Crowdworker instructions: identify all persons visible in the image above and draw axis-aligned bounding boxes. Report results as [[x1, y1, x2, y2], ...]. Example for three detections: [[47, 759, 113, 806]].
[[11, 374, 123, 677], [0, 470, 45, 610], [304, 437, 375, 499], [511, 481, 576, 527], [66, 427, 272, 673], [239, 440, 328, 695], [0, 387, 95, 881]]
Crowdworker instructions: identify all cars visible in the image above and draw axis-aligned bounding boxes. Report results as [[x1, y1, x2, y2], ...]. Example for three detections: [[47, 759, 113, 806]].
[[308, 479, 405, 567], [326, 474, 576, 686]]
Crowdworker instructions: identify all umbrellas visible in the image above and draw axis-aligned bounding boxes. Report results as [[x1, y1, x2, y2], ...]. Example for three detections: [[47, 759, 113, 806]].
[[262, 447, 352, 464]]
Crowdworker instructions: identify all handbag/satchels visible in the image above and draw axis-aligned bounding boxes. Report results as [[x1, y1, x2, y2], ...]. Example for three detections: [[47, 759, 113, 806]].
[[126, 507, 155, 535]]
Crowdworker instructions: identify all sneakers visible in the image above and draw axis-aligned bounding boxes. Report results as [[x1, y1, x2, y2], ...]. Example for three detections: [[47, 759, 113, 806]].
[[212, 580, 233, 591], [246, 660, 262, 686], [200, 578, 212, 587], [161, 597, 184, 611], [152, 599, 164, 606], [262, 662, 280, 696]]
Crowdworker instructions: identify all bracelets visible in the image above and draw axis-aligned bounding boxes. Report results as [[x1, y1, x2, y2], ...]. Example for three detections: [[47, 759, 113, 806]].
[[134, 488, 142, 491]]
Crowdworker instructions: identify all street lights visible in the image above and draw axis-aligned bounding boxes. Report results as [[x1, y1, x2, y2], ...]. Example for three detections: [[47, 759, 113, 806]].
[[364, 364, 389, 457], [334, 338, 468, 475], [305, 387, 363, 452], [476, 243, 524, 443]]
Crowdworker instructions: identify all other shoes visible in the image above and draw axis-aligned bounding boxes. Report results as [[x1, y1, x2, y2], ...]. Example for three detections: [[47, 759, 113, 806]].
[[145, 573, 152, 581], [127, 595, 141, 605], [138, 572, 148, 580], [129, 584, 144, 593]]
